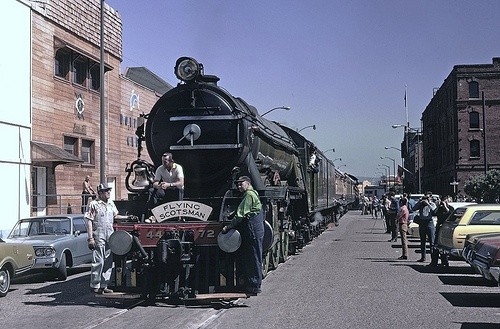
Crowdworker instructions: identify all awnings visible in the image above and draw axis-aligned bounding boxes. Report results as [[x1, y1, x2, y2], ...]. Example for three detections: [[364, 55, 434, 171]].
[[31, 141, 84, 168]]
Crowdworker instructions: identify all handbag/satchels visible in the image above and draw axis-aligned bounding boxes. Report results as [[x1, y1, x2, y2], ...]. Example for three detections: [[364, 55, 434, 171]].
[[413, 215, 429, 227]]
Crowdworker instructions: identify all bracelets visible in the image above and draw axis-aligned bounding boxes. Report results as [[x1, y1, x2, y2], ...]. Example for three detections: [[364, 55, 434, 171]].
[[170, 183, 172, 187]]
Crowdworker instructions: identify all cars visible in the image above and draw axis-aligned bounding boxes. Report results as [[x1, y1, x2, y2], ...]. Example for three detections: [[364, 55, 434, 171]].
[[434, 204, 500, 260], [391, 195, 477, 241], [0, 237, 38, 296], [4, 213, 93, 281], [461, 232, 500, 286]]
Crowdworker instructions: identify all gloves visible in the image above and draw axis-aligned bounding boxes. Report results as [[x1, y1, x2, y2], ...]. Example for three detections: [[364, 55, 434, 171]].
[[87, 238, 97, 250]]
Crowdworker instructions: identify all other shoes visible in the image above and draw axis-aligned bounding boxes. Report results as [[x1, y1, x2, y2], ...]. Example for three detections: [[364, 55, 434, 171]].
[[385, 230, 390, 233], [91, 288, 104, 294], [398, 255, 407, 260], [144, 216, 156, 224], [103, 288, 113, 293], [417, 259, 426, 261], [388, 238, 397, 242], [426, 264, 437, 268]]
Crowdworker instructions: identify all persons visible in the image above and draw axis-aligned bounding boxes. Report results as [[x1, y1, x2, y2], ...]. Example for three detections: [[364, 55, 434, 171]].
[[448, 195, 466, 202], [81, 175, 95, 214], [372, 196, 379, 219], [388, 191, 399, 242], [396, 198, 409, 260], [380, 193, 391, 234], [145, 153, 184, 223], [361, 195, 369, 215], [84, 184, 128, 294], [412, 191, 436, 262], [426, 196, 454, 267], [403, 193, 416, 219], [222, 176, 265, 293]]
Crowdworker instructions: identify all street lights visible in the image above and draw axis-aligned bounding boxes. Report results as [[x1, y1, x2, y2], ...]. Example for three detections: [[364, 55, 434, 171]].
[[375, 164, 390, 192], [392, 124, 421, 194], [380, 157, 395, 192], [385, 147, 404, 192]]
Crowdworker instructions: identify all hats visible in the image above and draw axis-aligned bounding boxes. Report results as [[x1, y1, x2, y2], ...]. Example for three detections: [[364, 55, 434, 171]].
[[155, 189, 165, 200], [234, 176, 251, 183], [96, 183, 112, 191]]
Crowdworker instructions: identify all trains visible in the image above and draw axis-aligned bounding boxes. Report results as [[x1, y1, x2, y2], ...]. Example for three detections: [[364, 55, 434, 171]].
[[94, 56, 361, 308]]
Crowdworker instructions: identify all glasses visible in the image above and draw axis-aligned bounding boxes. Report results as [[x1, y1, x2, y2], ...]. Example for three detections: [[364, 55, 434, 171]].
[[103, 189, 111, 191]]
